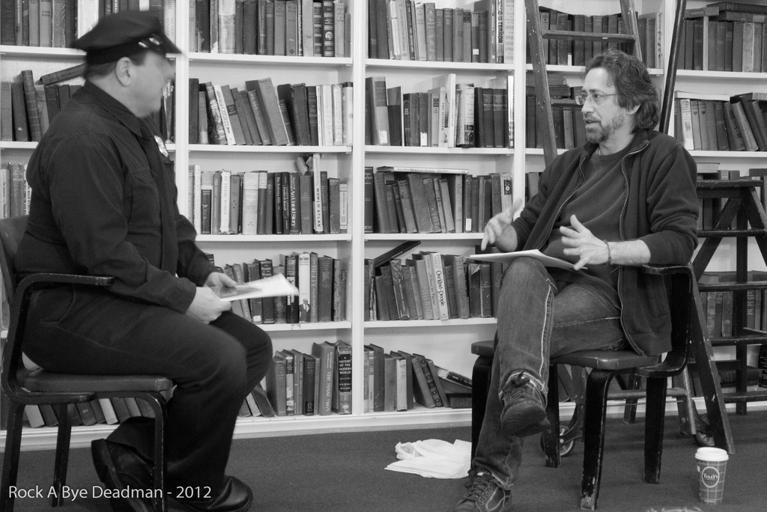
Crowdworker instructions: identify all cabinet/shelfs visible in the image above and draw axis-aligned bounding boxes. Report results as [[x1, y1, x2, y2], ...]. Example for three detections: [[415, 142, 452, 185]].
[[0, 0, 767, 449]]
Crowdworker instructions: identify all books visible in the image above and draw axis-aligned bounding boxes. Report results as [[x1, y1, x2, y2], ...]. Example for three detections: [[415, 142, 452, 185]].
[[1, 2, 766, 430]]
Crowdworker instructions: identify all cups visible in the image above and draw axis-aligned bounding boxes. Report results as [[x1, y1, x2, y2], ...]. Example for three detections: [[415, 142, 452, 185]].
[[694, 449, 730, 503]]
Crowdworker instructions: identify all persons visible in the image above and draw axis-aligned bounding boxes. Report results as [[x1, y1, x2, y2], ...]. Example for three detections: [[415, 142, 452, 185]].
[[452, 49, 701, 512], [14, 10, 274, 512]]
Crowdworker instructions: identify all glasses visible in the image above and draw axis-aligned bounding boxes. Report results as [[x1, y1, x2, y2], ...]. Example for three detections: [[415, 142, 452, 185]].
[[574, 94, 619, 105]]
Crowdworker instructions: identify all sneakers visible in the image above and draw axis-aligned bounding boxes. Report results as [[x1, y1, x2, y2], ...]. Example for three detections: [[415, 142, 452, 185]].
[[497, 367, 551, 438], [454, 470, 513, 512]]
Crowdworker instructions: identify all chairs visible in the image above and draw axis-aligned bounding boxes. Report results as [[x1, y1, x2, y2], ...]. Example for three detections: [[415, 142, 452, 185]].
[[0, 218, 174, 512], [472, 262, 693, 512]]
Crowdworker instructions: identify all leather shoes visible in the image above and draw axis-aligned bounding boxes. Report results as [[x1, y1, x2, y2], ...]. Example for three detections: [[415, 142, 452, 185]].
[[167, 475, 253, 511], [91, 439, 159, 510]]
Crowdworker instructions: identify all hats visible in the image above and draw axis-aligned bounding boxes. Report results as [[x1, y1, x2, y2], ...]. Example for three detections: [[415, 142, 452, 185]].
[[71, 8, 182, 65]]
